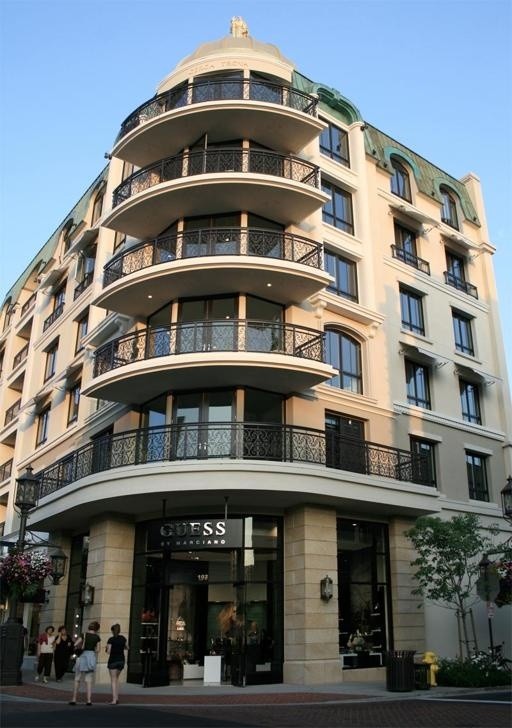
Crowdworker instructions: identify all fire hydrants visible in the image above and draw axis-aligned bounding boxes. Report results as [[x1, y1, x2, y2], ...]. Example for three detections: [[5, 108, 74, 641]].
[[422, 651, 438, 687]]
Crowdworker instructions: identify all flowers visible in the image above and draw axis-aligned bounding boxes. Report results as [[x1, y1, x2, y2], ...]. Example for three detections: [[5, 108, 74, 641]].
[[1, 542, 52, 590]]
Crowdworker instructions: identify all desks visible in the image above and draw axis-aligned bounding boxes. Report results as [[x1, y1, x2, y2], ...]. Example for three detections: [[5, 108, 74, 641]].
[[340, 653, 383, 668]]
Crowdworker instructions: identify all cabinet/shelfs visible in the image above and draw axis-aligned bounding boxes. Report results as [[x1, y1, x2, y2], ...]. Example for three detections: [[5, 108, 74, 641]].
[[138, 620, 159, 655]]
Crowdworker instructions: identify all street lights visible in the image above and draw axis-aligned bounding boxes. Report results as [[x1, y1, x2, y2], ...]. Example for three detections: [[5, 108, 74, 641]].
[[0, 466, 42, 687], [501, 475, 512, 527], [478, 553, 494, 663]]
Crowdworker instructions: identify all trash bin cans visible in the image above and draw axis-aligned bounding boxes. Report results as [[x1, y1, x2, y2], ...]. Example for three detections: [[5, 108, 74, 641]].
[[385, 650, 416, 691]]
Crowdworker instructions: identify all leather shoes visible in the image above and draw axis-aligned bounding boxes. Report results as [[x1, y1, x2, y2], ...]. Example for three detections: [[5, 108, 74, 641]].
[[86, 700, 92, 705], [68, 700, 76, 705]]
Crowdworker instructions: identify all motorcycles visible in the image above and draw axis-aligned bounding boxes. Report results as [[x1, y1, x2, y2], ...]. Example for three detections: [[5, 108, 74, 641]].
[[471, 642, 512, 671]]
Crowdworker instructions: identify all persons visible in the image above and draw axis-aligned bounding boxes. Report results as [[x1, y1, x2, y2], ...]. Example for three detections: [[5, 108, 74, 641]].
[[218, 601, 260, 636], [18, 618, 129, 705], [222, 631, 231, 682]]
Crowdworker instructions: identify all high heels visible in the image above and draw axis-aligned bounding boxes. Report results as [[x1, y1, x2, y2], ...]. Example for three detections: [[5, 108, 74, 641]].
[[109, 700, 119, 704]]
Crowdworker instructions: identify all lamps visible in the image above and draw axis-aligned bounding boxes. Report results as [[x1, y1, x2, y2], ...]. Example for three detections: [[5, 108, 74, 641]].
[[320, 575, 333, 602], [81, 584, 94, 606]]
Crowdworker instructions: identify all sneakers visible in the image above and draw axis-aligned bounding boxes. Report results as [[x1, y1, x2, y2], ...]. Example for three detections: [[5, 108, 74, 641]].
[[35, 675, 48, 683]]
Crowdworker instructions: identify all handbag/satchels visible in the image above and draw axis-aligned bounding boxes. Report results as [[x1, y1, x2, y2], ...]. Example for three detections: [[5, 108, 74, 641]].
[[75, 647, 83, 656]]
